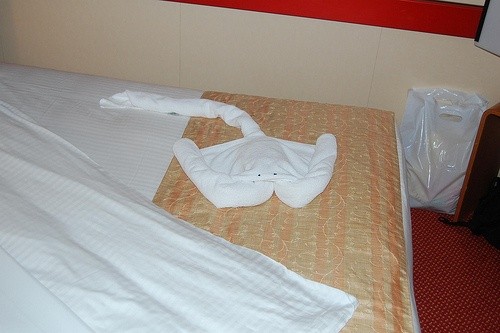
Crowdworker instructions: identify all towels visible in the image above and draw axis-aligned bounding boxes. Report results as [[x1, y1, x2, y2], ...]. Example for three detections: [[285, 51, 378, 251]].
[[98, 88, 339, 209]]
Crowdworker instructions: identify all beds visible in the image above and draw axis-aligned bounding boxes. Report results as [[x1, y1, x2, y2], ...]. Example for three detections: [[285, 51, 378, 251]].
[[1, 61, 422, 332]]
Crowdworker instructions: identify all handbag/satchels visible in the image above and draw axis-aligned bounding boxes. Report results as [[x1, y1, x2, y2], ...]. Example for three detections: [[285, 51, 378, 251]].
[[397, 86, 492, 216]]
